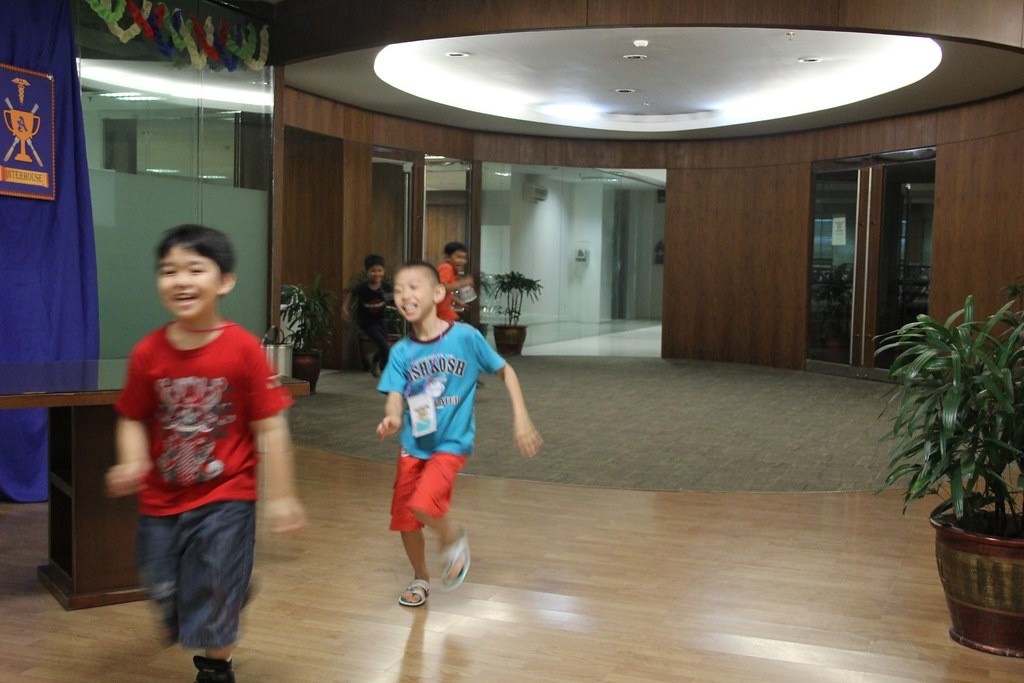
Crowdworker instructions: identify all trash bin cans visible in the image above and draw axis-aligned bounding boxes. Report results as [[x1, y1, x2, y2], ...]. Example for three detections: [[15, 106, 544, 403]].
[[265, 343, 293, 379], [281, 285, 304, 349]]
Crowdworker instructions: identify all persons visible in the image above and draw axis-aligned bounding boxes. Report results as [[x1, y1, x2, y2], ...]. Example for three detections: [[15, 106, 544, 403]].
[[433, 241, 483, 389], [375, 261, 543, 605], [106, 224, 301, 683], [341, 253, 395, 377]]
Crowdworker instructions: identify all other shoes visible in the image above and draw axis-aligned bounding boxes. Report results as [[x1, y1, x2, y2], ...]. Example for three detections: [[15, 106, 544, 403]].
[[476, 381, 483, 387], [370, 352, 382, 378], [192, 656, 236, 683]]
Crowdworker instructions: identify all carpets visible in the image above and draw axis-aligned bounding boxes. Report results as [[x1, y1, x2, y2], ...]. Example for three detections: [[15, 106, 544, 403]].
[[285, 355, 953, 494]]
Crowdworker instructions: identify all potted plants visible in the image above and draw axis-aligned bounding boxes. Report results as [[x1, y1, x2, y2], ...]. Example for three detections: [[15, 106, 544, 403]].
[[280, 273, 339, 394], [347, 271, 405, 372], [871, 284, 1024, 659], [476, 271, 544, 355]]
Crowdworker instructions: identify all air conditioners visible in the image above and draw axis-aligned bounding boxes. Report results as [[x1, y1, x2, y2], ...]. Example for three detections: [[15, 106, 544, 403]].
[[521, 182, 548, 202]]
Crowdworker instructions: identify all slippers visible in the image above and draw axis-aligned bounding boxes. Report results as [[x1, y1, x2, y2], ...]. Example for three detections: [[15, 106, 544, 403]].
[[399, 578, 431, 606], [439, 527, 470, 590]]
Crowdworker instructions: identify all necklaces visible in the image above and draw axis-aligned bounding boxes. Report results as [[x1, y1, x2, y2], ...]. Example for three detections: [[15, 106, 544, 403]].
[[176, 321, 238, 334]]
